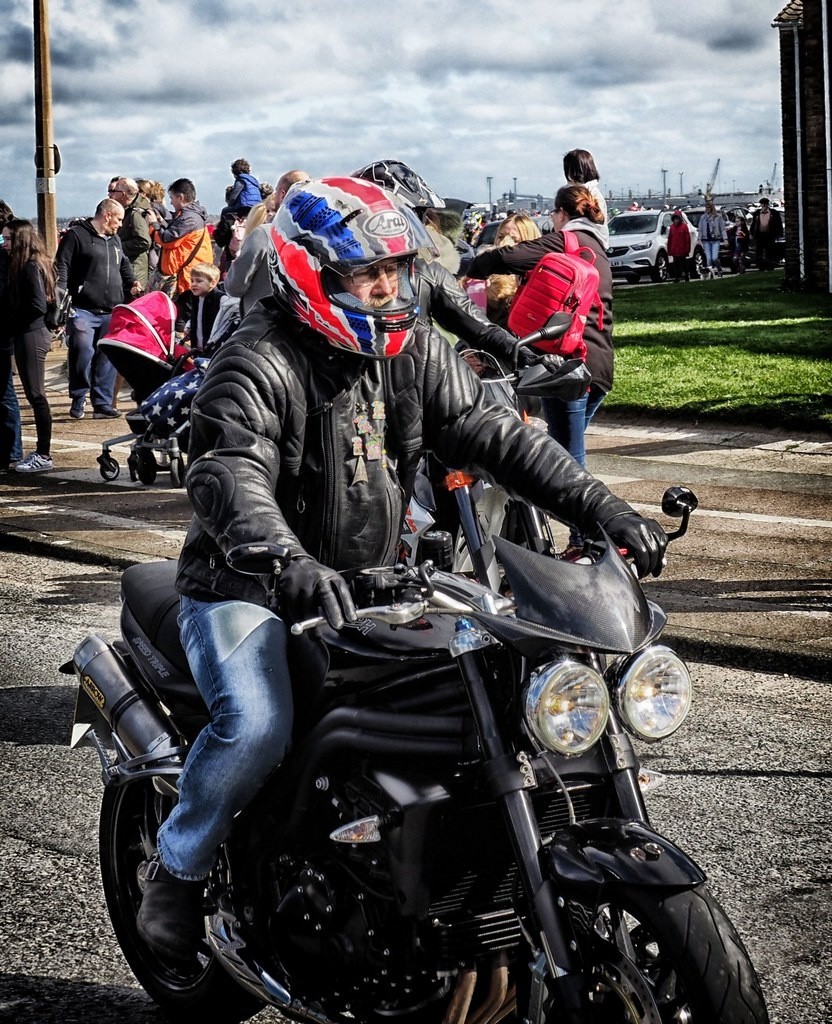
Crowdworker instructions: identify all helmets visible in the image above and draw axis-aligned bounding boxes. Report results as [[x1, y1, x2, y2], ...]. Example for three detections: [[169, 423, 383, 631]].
[[267, 179, 440, 359], [462, 217, 478, 233], [470, 212, 482, 225], [351, 159, 446, 223]]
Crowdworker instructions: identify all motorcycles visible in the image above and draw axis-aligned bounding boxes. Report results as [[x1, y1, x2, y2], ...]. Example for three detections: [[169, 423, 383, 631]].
[[416, 309, 593, 602], [61, 487, 771, 1024]]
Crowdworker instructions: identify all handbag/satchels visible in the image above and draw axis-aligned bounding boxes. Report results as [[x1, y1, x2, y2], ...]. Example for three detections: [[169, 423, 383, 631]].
[[143, 270, 178, 303]]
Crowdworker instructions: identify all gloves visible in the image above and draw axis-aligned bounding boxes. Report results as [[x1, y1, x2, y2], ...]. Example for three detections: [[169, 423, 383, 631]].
[[269, 556, 357, 630], [599, 513, 672, 580]]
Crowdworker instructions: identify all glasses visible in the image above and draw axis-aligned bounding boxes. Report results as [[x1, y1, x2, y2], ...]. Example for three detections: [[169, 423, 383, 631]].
[[324, 261, 408, 289], [108, 188, 123, 194], [548, 207, 561, 219]]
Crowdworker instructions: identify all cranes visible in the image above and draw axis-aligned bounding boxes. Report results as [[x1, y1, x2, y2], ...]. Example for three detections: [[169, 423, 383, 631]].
[[704, 158, 722, 204]]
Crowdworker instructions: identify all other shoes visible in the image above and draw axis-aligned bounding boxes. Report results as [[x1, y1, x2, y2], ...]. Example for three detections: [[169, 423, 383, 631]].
[[718, 267, 723, 277]]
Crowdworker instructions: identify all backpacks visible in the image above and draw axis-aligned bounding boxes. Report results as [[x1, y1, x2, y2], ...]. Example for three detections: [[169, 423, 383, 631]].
[[45, 285, 72, 330], [506, 231, 605, 351], [140, 356, 212, 437], [228, 211, 247, 256]]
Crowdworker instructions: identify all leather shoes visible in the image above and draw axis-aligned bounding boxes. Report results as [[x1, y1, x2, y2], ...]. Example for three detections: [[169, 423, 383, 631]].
[[135, 854, 204, 956]]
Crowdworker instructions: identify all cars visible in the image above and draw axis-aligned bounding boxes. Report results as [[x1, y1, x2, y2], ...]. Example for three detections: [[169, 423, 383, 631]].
[[474, 217, 553, 250], [748, 206, 786, 265]]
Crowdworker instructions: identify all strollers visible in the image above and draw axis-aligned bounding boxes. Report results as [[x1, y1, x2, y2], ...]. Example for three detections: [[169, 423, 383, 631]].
[[97, 358, 209, 488], [100, 290, 208, 410]]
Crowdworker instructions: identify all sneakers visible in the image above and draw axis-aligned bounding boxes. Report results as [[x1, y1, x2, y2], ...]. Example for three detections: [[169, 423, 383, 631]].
[[92, 405, 121, 419], [0, 457, 22, 475], [68, 399, 87, 420], [15, 451, 52, 472]]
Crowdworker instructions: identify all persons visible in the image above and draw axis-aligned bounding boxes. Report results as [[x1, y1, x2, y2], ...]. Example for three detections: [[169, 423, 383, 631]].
[[0, 198, 51, 473], [732, 198, 783, 275], [697, 203, 728, 282], [172, 265, 230, 358], [668, 210, 692, 281], [52, 198, 145, 419], [106, 159, 308, 319], [138, 148, 666, 958], [628, 200, 691, 212]]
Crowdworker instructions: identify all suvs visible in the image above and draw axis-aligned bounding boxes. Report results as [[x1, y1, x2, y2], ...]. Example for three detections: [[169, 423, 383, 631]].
[[683, 203, 761, 270], [608, 209, 706, 285]]
